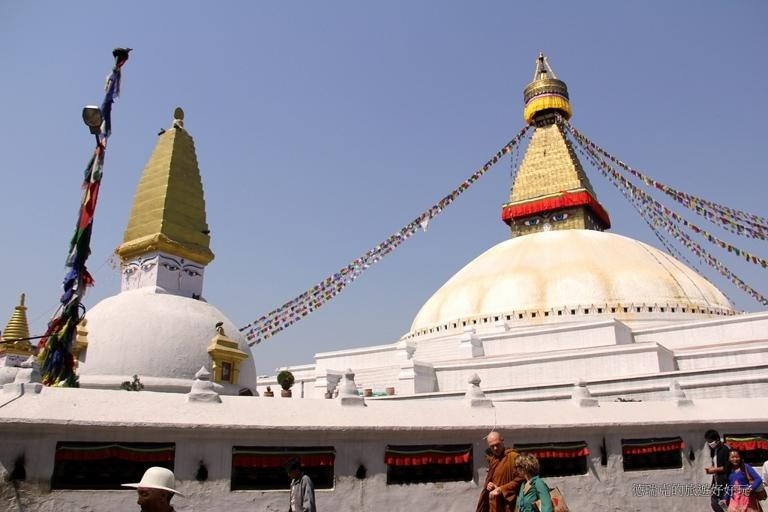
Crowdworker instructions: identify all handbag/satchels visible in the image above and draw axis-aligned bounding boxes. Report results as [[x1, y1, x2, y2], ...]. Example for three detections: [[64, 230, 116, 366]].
[[750, 480, 768, 501], [535, 487, 568, 512]]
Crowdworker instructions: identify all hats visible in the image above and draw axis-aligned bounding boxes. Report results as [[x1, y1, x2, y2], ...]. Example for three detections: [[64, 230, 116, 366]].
[[122, 467, 184, 499]]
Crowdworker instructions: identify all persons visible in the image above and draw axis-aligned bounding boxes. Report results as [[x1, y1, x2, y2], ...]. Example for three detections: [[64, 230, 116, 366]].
[[718, 448, 763, 512], [483, 448, 494, 463], [705, 429, 733, 512], [284, 458, 316, 512], [121, 467, 185, 512], [476, 432, 526, 512], [514, 453, 553, 512]]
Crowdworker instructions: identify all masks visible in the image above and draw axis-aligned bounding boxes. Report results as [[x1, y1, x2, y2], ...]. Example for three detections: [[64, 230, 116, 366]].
[[708, 441, 717, 448]]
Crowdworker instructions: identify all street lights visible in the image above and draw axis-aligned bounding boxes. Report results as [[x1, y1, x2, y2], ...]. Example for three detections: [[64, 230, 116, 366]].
[[35, 46, 132, 386]]
